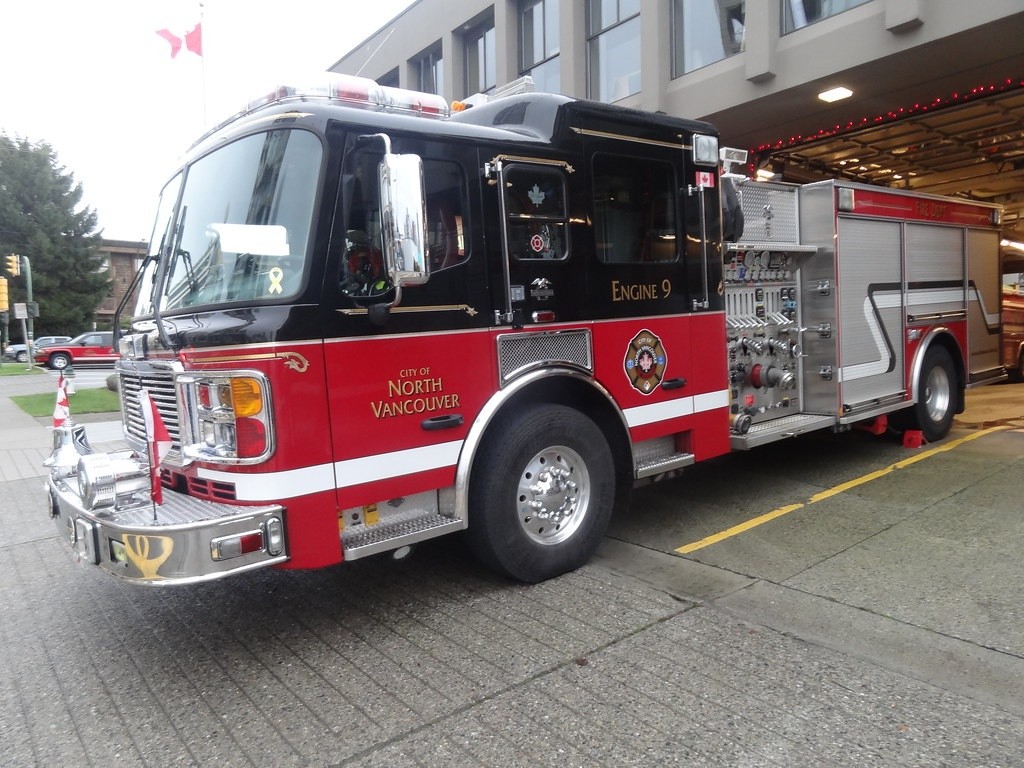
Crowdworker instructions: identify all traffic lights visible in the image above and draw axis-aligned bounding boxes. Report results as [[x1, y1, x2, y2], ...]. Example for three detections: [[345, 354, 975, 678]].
[[5, 252, 20, 277]]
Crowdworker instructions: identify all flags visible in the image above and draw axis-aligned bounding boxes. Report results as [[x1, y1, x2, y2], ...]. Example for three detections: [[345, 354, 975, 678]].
[[151, 11, 204, 62]]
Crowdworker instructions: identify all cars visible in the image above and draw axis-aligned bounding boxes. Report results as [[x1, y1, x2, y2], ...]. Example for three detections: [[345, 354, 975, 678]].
[[3, 330, 129, 370]]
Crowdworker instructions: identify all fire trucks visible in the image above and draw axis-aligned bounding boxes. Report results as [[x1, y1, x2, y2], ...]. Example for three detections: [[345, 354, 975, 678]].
[[39, 69, 1009, 587]]
